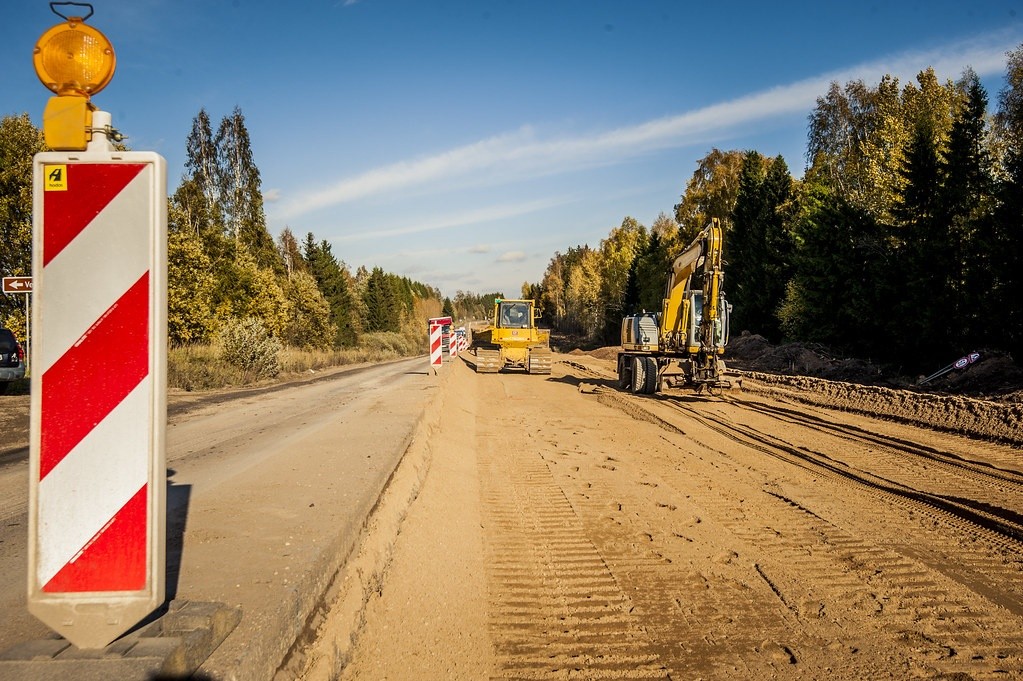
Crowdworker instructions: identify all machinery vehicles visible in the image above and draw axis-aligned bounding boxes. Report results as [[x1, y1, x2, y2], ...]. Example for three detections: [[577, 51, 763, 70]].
[[468, 296, 552, 375], [616, 218, 734, 397]]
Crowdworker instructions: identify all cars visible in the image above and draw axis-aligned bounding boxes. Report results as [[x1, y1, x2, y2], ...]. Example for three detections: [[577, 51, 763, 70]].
[[0, 327, 26, 391]]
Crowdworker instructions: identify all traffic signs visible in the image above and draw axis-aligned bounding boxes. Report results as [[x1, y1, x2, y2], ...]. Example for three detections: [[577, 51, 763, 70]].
[[2, 277, 33, 293]]
